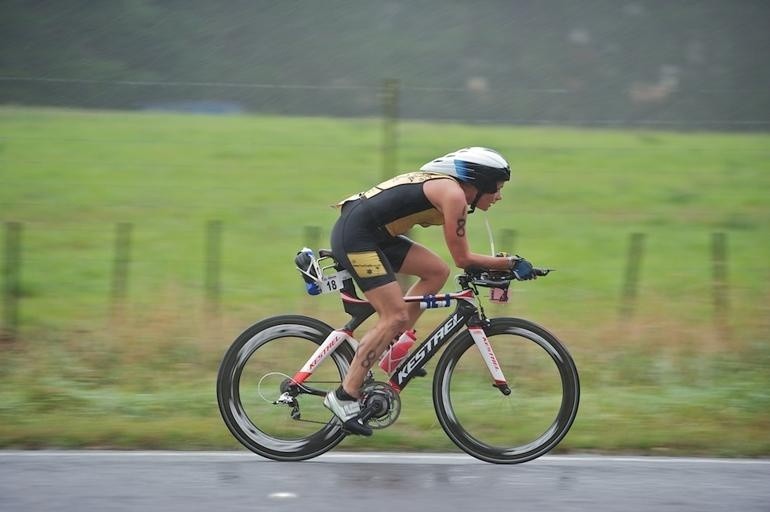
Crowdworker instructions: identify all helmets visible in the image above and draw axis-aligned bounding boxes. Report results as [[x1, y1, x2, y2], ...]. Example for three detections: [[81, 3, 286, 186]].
[[418, 146, 511, 192]]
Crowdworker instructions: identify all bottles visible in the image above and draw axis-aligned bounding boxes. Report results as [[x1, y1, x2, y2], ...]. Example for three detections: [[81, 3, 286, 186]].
[[378, 328, 418, 375], [294, 251, 323, 296]]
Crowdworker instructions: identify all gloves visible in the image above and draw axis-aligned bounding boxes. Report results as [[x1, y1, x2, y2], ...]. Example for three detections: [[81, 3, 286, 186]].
[[511, 255, 536, 281]]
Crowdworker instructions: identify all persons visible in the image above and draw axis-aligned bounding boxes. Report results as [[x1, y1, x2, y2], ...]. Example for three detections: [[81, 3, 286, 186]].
[[319, 144, 538, 440]]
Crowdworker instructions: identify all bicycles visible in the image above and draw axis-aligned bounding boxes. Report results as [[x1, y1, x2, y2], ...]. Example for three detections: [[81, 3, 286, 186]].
[[213, 246, 581, 465]]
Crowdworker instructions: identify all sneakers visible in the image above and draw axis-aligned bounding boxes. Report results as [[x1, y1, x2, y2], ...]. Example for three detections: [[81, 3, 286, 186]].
[[323, 389, 373, 436], [380, 341, 427, 377]]
